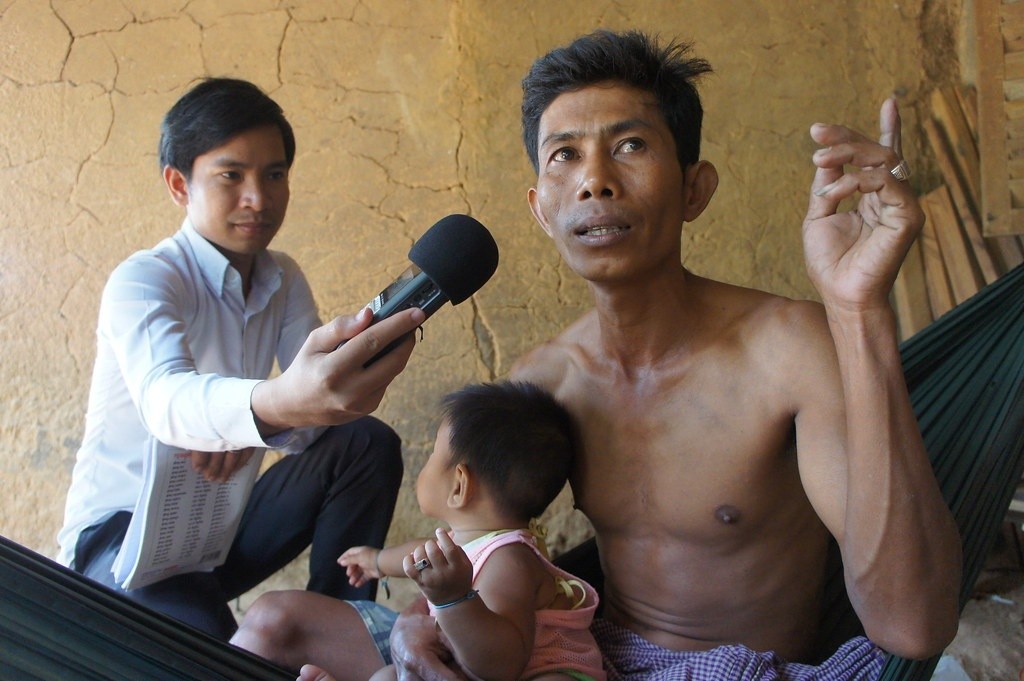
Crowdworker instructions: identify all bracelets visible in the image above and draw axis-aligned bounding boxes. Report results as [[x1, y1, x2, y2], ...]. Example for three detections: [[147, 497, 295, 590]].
[[375, 547, 390, 599], [434, 589, 479, 609]]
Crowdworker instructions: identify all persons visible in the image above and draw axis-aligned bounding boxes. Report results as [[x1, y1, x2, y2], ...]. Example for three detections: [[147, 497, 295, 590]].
[[55, 76, 404, 641], [227, 29, 963, 681], [296, 378, 604, 681]]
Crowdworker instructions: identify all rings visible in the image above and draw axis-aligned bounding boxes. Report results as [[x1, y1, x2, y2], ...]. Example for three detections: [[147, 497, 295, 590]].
[[414, 557, 430, 570], [891, 160, 911, 182]]
